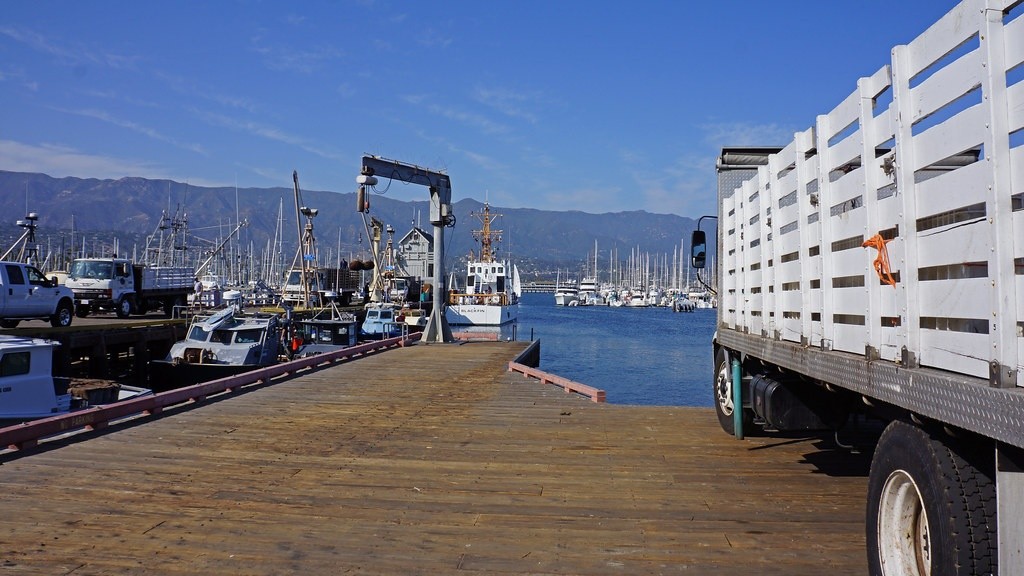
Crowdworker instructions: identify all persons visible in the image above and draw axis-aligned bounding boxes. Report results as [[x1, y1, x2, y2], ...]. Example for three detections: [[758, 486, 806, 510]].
[[281, 313, 297, 363], [363, 280, 373, 306], [396, 312, 406, 326], [189, 278, 203, 311]]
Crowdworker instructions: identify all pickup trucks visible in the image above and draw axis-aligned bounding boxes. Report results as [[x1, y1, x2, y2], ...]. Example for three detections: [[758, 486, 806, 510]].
[[1, 260, 76, 328]]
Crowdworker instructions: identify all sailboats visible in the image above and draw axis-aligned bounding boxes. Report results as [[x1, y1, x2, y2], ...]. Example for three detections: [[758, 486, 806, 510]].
[[554, 238, 718, 313], [0, 170, 367, 310]]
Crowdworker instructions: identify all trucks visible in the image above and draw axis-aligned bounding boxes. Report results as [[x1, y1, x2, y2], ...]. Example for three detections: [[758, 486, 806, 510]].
[[63, 256, 195, 319], [385, 276, 420, 301], [281, 268, 359, 307], [691, 0, 1024, 576]]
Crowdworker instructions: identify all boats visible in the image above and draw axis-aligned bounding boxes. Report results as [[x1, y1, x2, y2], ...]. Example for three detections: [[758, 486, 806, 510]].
[[0, 334, 152, 426], [395, 300, 430, 333], [149, 288, 302, 381], [290, 287, 363, 361], [359, 307, 408, 339], [444, 187, 518, 326]]
